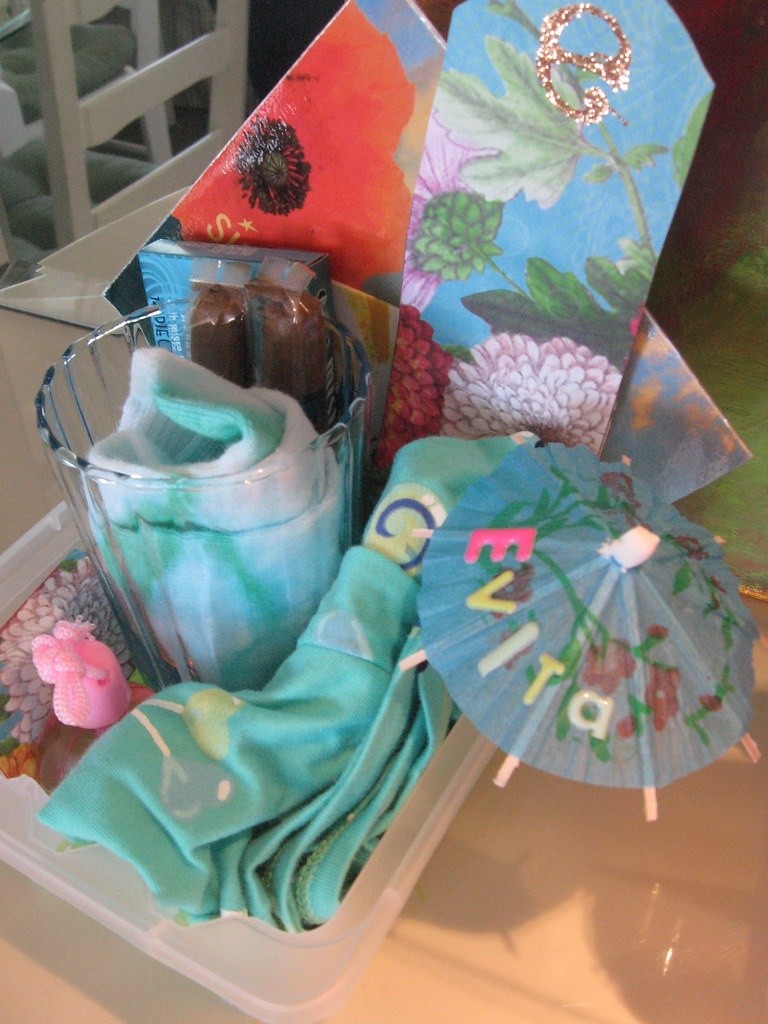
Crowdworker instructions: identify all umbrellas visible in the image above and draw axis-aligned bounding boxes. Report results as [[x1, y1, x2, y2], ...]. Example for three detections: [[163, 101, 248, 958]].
[[399, 455, 768, 821]]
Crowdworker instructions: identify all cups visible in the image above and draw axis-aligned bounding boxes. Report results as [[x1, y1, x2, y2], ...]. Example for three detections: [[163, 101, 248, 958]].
[[34, 298, 372, 692]]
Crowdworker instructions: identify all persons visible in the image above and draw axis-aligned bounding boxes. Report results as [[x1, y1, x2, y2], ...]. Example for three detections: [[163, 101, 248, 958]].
[[206, 1, 767, 321]]
